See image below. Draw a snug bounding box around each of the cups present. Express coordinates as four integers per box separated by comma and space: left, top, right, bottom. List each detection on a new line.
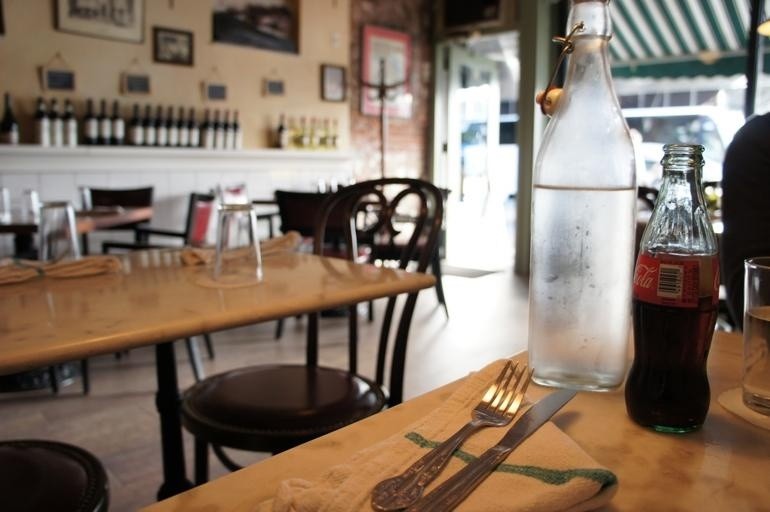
213, 204, 262, 288
37, 204, 80, 265
75, 185, 93, 213
1, 187, 40, 226
743, 257, 770, 418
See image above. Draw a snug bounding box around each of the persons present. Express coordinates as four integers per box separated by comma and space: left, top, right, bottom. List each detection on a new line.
719, 110, 770, 342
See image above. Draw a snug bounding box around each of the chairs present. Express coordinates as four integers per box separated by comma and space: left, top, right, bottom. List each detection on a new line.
174, 185, 446, 496
0, 436, 114, 512
3, 180, 446, 392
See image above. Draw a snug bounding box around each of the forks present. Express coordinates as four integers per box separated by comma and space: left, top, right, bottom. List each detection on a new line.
369, 355, 534, 512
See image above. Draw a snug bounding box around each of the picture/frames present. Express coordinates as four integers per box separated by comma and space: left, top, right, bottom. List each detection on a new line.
321, 64, 346, 101
201, 83, 227, 102
42, 66, 75, 92
123, 73, 152, 93
263, 79, 285, 95
58, 1, 145, 44
152, 26, 192, 65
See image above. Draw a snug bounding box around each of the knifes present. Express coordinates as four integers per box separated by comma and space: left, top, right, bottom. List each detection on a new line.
408, 388, 583, 512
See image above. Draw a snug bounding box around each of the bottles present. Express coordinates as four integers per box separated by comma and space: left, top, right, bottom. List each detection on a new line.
626, 145, 719, 439
1, 94, 341, 147
529, 0, 638, 396
